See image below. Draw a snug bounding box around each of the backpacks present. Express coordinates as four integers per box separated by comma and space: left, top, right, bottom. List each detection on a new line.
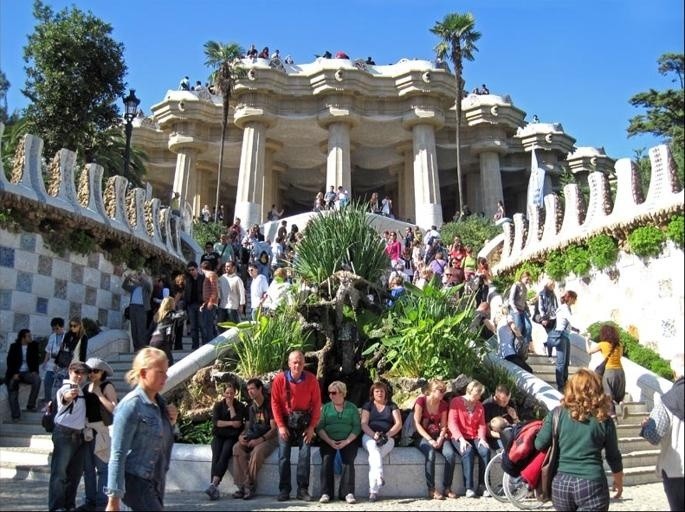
42, 383, 73, 432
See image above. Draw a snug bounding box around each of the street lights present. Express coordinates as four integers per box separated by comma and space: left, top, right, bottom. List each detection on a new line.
121, 88, 140, 182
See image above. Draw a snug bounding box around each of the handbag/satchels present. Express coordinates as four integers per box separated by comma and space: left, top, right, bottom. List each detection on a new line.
400, 411, 417, 447
547, 330, 561, 346
54, 349, 72, 368
533, 310, 546, 322
517, 342, 528, 360
541, 444, 557, 499
595, 358, 608, 376
100, 399, 120, 426
288, 411, 311, 428
216, 416, 243, 437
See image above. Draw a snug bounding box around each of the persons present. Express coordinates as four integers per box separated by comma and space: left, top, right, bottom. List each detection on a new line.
48, 346, 177, 512
122, 193, 313, 366
314, 50, 376, 66
452, 201, 506, 224
386, 224, 684, 512
532, 115, 539, 124
178, 76, 215, 93
314, 186, 412, 224
473, 83, 490, 94
246, 46, 294, 65
5, 317, 88, 422
204, 350, 402, 504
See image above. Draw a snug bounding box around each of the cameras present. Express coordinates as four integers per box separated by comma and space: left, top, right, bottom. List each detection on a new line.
377, 434, 387, 445
71, 384, 78, 389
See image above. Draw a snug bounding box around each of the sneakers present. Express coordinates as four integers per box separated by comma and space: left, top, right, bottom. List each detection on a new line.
319, 494, 329, 502
368, 494, 376, 502
466, 489, 475, 497
277, 491, 289, 501
232, 488, 253, 500
205, 485, 219, 500
444, 489, 456, 498
483, 490, 491, 496
429, 488, 442, 499
345, 493, 355, 504
297, 491, 311, 500
377, 478, 384, 488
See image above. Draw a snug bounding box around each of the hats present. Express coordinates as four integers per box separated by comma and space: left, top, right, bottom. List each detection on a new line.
85, 358, 113, 376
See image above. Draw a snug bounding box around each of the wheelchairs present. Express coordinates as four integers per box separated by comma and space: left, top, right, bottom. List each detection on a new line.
484, 421, 547, 510
